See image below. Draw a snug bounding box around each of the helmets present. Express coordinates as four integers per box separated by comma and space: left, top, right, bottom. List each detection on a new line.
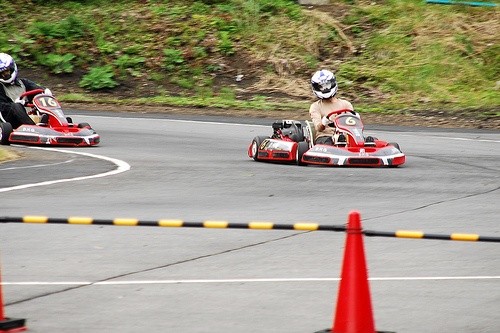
0, 51, 18, 84
312, 69, 339, 98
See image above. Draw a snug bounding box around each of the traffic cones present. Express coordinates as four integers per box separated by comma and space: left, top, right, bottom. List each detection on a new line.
311, 211, 396, 333
0, 257, 28, 333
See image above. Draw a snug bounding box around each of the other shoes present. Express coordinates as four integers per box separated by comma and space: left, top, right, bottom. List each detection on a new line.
366, 137, 374, 147
38, 113, 47, 127
66, 116, 72, 126
338, 135, 346, 147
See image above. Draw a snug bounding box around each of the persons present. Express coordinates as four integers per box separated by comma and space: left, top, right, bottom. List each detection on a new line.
0, 52, 73, 129
309, 69, 379, 146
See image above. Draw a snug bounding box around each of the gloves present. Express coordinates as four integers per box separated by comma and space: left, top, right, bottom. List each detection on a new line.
356, 112, 361, 118
321, 115, 329, 126
15, 97, 25, 105
42, 87, 52, 96
